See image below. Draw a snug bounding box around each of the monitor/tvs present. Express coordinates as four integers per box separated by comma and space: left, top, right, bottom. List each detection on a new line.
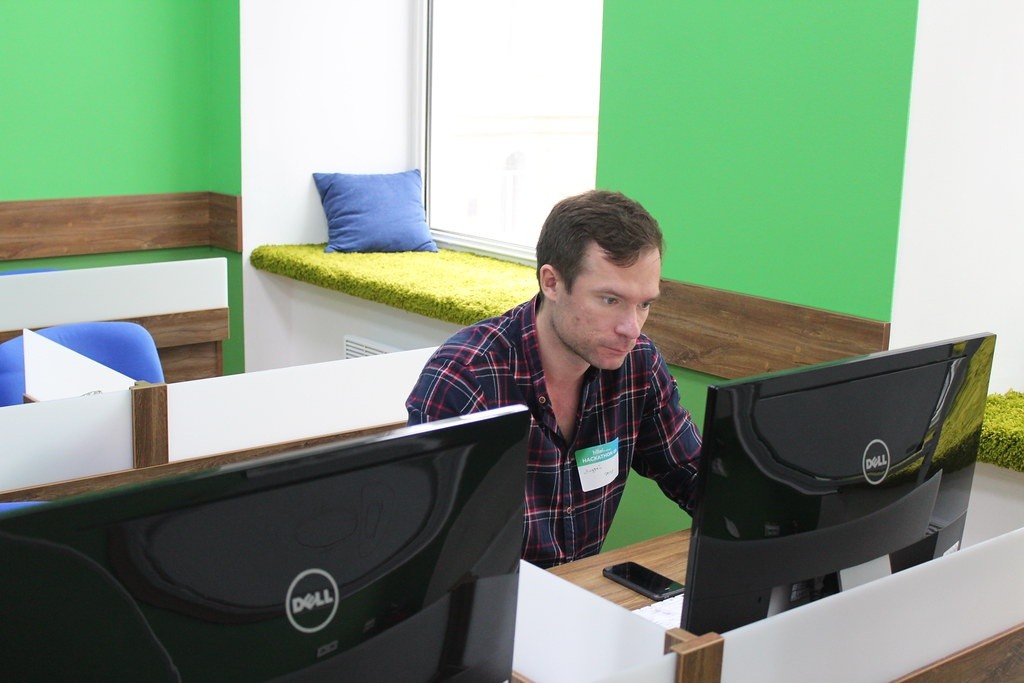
0, 403, 531, 683
678, 331, 997, 638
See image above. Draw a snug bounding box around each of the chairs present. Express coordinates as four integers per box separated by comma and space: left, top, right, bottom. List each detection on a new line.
1, 316, 169, 408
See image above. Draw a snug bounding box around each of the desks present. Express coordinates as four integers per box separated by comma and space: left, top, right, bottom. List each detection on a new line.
3, 335, 480, 509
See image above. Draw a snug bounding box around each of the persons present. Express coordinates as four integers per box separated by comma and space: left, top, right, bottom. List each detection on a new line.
400, 185, 706, 570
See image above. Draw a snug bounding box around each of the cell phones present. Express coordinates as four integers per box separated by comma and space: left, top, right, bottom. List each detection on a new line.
603, 561, 685, 602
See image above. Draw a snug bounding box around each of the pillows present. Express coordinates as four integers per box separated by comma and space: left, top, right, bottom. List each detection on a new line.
311, 166, 440, 258
